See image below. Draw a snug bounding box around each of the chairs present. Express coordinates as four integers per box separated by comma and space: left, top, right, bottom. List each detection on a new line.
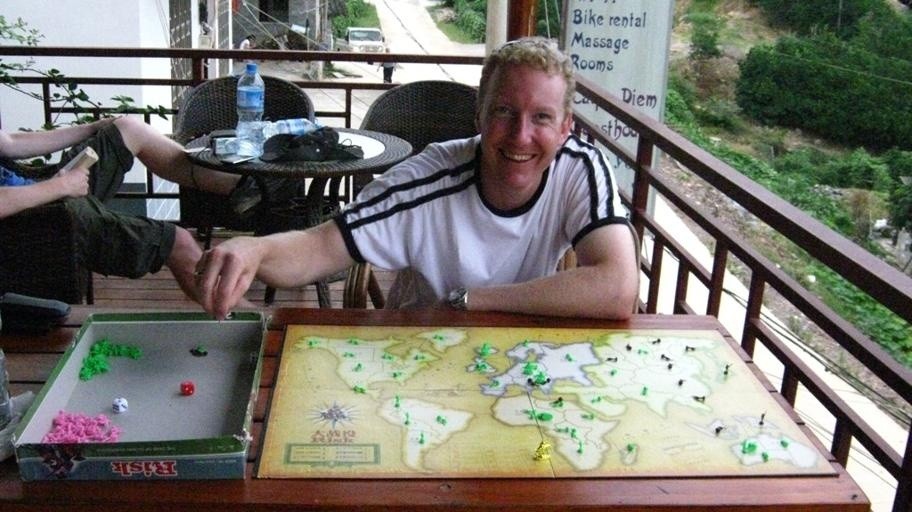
174, 75, 314, 248
351, 79, 477, 202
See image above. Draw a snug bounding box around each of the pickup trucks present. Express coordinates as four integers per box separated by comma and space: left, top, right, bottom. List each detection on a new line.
335, 27, 385, 53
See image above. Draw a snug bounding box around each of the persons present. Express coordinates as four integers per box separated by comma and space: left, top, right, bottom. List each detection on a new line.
1, 111, 260, 310
1, 312, 35, 462
187, 37, 647, 323
375, 47, 396, 82
239, 34, 256, 62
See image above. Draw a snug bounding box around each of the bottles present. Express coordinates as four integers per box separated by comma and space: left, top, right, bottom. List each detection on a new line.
235, 63, 265, 157
262, 118, 323, 144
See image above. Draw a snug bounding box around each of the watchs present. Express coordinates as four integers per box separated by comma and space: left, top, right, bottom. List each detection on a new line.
448, 287, 468, 310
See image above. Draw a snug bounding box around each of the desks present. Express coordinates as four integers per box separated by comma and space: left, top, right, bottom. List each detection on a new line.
182, 126, 413, 308
1, 305, 869, 509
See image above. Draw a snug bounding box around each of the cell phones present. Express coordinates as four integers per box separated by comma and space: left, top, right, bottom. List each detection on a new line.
220, 155, 255, 164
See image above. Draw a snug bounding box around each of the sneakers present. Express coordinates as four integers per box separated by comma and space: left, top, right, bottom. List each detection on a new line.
228, 175, 288, 217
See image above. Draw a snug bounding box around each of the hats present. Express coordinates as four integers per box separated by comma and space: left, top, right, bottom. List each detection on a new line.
258, 127, 365, 162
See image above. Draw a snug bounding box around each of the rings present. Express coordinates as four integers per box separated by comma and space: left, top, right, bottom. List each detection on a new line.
193, 268, 205, 279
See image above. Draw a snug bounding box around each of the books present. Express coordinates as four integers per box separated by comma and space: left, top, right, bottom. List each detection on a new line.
51, 146, 98, 179
